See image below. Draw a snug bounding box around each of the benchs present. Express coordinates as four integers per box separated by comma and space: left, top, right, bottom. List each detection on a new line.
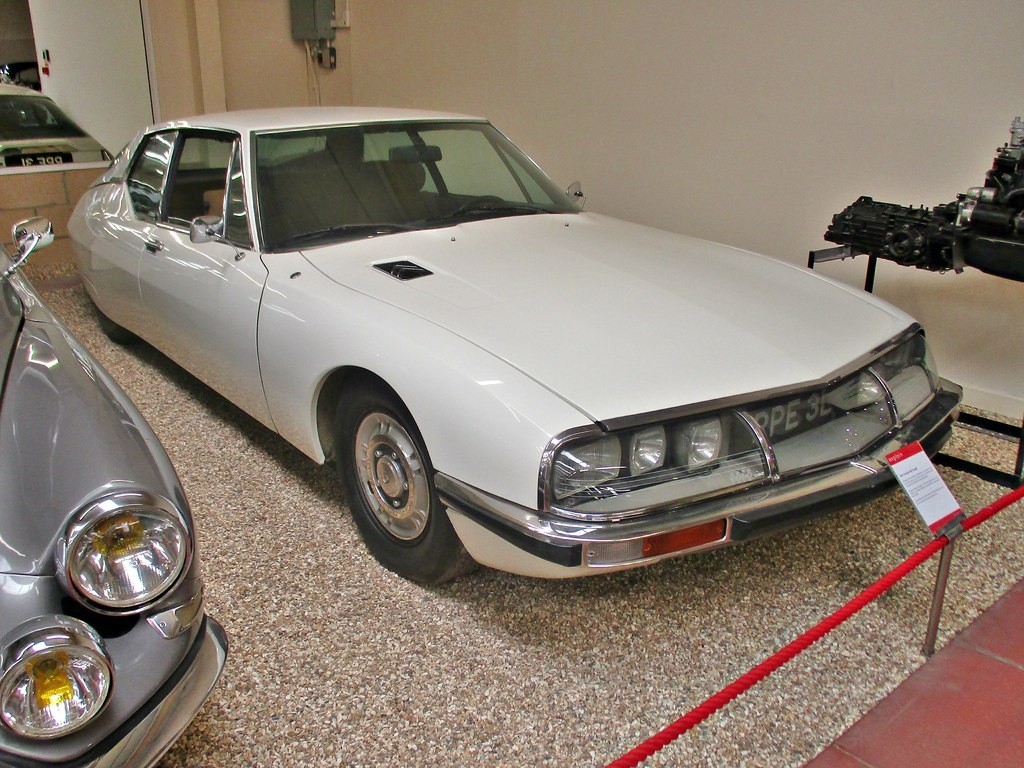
204, 159, 433, 246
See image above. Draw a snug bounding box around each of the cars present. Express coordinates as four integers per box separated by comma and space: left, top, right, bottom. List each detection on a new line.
67, 107, 965, 587
0, 83, 115, 167
0, 216, 228, 768
